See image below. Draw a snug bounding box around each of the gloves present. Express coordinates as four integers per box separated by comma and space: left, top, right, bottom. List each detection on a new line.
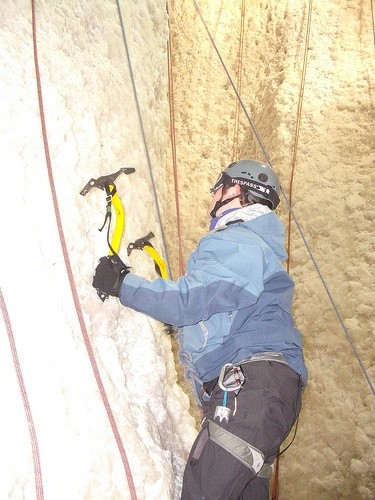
93, 256, 122, 297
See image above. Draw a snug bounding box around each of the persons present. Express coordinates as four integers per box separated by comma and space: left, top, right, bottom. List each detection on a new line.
92, 159, 308, 500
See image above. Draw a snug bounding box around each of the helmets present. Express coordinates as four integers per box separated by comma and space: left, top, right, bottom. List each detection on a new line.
211, 160, 280, 207
